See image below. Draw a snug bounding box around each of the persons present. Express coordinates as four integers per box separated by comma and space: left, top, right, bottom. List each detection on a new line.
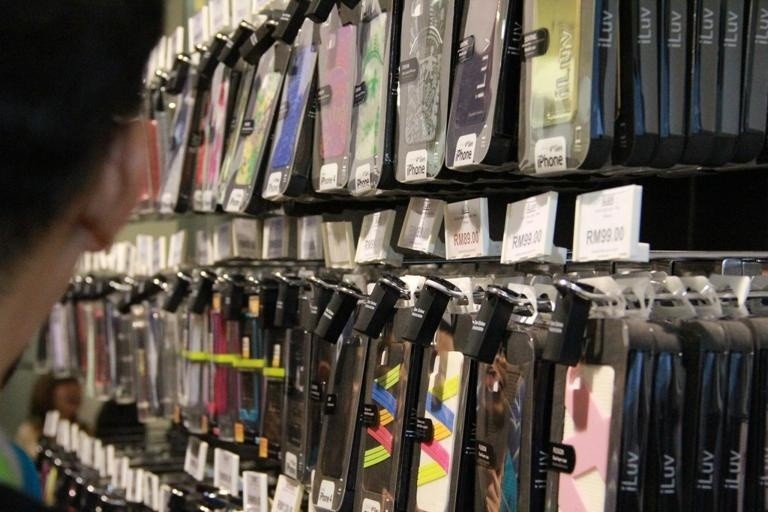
0, 0, 163, 511
